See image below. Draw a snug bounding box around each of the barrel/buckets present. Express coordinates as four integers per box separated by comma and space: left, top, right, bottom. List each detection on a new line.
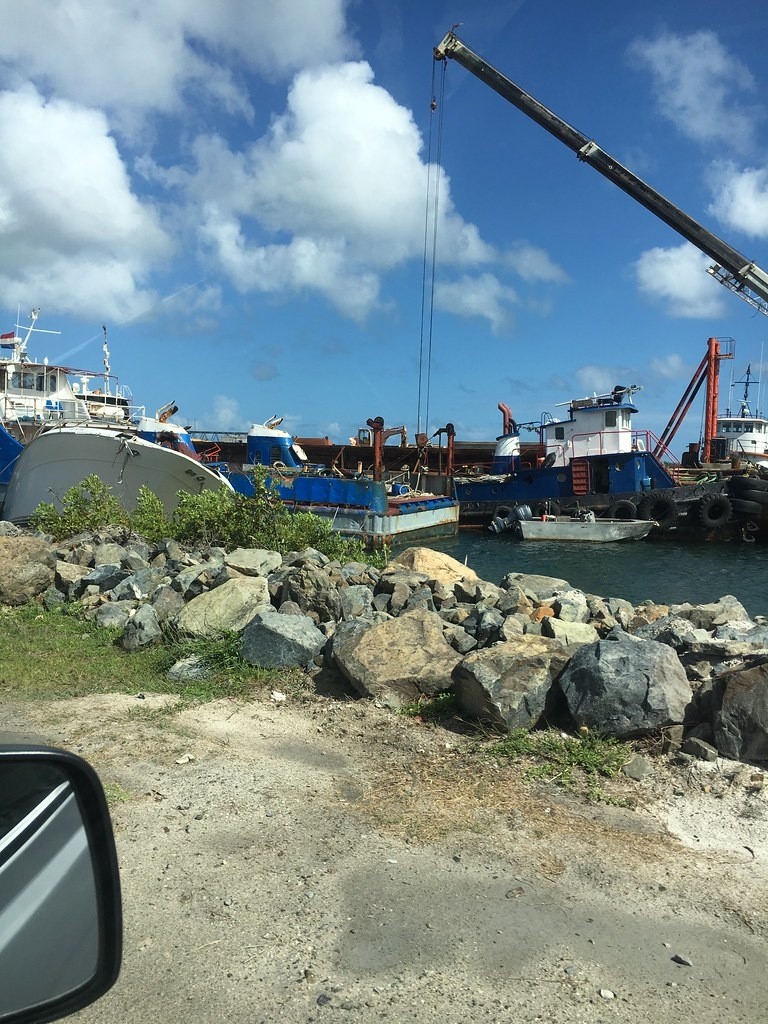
401, 483, 409, 494
732, 458, 740, 469
391, 484, 401, 496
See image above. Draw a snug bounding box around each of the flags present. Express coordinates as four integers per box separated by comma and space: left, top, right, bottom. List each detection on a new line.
0, 332, 15, 349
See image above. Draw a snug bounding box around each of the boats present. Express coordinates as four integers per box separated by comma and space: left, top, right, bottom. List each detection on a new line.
214, 415, 461, 551
700, 360, 768, 475
488, 505, 656, 544
452, 382, 732, 533
0, 418, 238, 527
0, 300, 221, 511
62, 322, 548, 471
